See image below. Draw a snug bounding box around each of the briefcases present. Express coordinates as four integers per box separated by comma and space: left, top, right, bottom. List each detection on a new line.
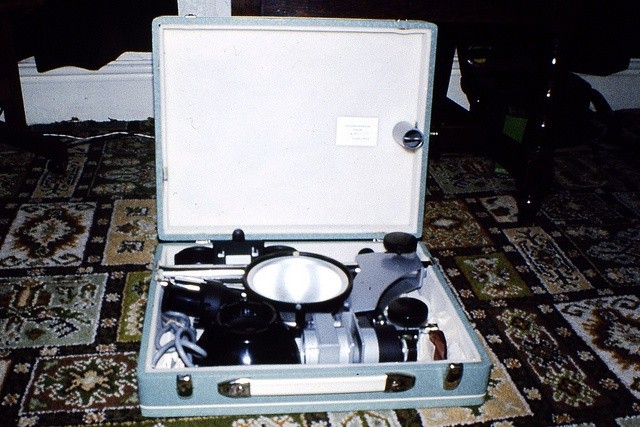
138, 14, 492, 418
